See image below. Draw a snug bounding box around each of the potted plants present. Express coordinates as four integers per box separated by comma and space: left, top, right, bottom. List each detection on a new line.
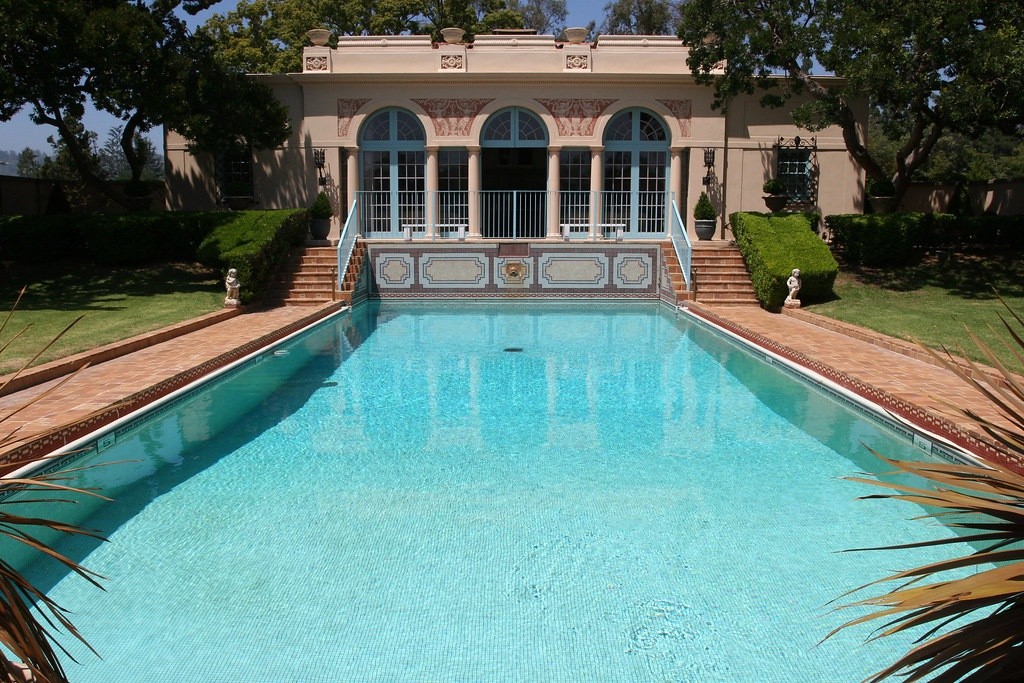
307, 190, 334, 240
762, 179, 789, 213
694, 191, 717, 241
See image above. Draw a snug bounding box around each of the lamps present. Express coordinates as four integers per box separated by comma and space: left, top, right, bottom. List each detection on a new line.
702, 148, 714, 185
616, 230, 623, 240
314, 148, 326, 185
458, 226, 465, 240
404, 227, 413, 241
562, 224, 570, 242
